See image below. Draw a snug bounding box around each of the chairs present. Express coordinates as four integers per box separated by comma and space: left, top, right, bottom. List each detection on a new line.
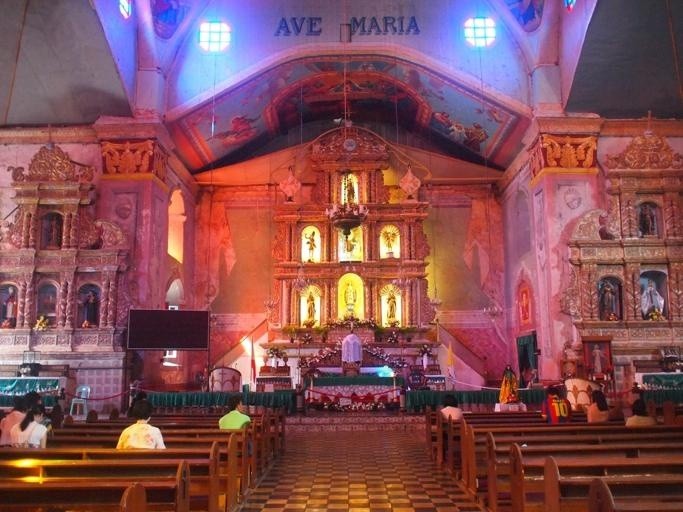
70, 385, 91, 414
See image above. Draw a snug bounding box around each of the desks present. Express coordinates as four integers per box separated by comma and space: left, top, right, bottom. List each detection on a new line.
402, 387, 564, 412
300, 375, 404, 411
144, 390, 298, 416
0, 376, 67, 410
640, 373, 683, 402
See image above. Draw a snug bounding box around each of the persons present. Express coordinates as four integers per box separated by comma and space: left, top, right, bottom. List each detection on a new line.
542, 385, 656, 427
114, 402, 160, 452
218, 396, 253, 457
0, 391, 55, 449
439, 396, 463, 421
126, 392, 147, 416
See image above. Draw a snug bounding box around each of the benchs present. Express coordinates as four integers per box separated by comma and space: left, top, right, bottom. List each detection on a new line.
417, 402, 683, 512
0, 405, 285, 510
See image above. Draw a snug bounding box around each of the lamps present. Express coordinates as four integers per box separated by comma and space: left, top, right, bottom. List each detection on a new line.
389, 1, 411, 294
259, 1, 288, 315
462, 2, 505, 324
327, 1, 370, 239
425, 2, 445, 309
289, 1, 314, 300
